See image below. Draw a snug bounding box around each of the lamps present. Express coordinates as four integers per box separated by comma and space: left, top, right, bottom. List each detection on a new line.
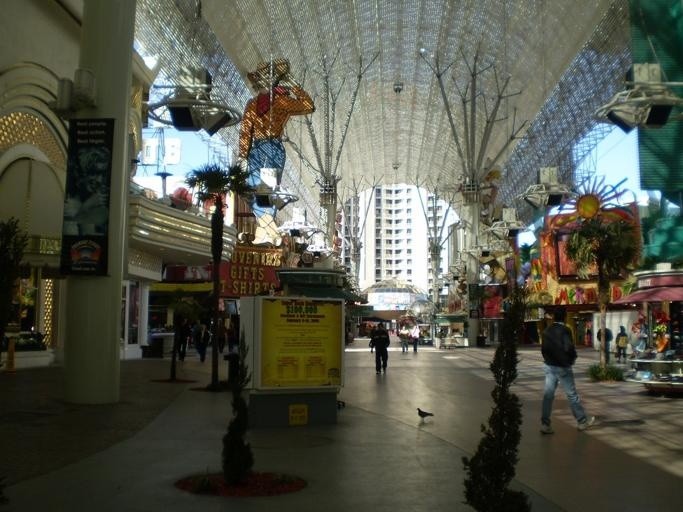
145, 66, 242, 136
245, 180, 334, 260
448, 165, 579, 283
47, 65, 99, 115
594, 61, 683, 134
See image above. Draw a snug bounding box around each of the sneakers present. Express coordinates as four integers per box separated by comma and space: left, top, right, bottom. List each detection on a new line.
539, 423, 553, 434
577, 416, 595, 431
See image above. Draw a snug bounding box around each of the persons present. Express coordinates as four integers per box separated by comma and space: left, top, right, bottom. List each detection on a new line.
597, 327, 613, 363
177, 318, 236, 365
370, 325, 377, 353
369, 322, 390, 375
399, 325, 409, 353
238, 56, 316, 247
631, 313, 683, 359
408, 325, 420, 352
615, 325, 630, 364
62, 142, 111, 236
540, 309, 597, 435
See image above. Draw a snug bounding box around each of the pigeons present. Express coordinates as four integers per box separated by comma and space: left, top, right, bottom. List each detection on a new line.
416, 408, 434, 421
